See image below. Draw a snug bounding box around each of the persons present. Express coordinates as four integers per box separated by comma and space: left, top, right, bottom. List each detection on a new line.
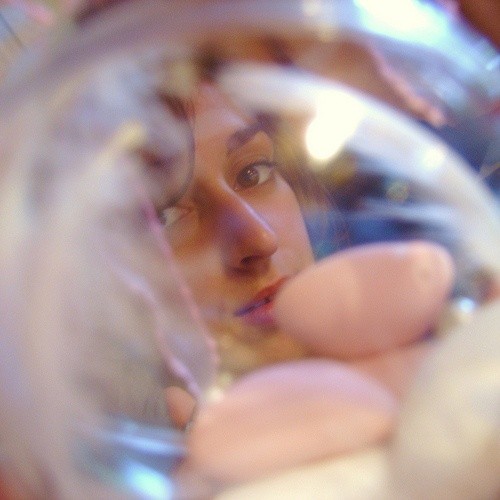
33, 51, 500, 500
